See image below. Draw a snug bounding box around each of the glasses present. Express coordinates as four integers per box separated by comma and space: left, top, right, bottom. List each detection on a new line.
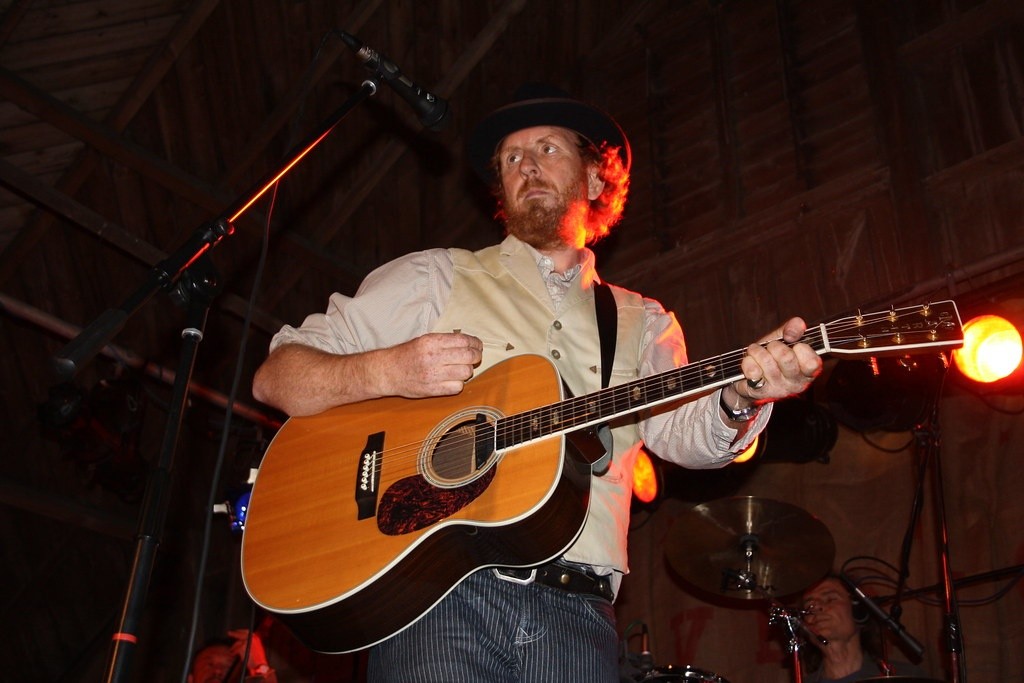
800, 592, 860, 610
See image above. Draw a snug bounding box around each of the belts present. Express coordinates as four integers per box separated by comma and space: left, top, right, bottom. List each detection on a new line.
492, 557, 614, 603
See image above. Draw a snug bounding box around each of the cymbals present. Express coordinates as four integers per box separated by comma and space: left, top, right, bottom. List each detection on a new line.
659, 497, 837, 601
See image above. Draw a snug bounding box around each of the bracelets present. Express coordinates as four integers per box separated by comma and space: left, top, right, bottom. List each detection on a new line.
249, 664, 271, 676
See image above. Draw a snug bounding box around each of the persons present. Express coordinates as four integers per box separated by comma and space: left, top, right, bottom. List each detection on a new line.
799, 568, 931, 683
187, 627, 278, 683
252, 85, 825, 683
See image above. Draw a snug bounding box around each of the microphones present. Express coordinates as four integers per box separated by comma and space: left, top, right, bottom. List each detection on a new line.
639, 624, 654, 672
335, 30, 452, 129
842, 574, 925, 657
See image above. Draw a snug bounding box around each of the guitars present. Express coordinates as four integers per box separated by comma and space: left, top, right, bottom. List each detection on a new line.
238, 301, 968, 659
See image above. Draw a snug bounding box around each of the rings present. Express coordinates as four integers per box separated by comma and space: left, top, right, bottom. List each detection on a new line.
747, 378, 766, 389
806, 376, 816, 381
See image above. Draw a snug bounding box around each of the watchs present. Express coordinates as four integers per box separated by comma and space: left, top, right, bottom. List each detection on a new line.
720, 396, 757, 422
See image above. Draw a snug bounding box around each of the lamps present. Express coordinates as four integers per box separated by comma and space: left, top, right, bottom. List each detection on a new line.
632, 314, 1024, 506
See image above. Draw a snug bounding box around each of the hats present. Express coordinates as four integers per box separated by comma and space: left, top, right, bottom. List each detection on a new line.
465, 85, 631, 226
